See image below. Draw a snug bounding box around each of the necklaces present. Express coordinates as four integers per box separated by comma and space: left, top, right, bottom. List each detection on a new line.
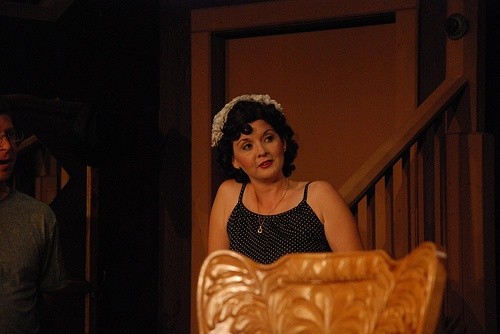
257, 178, 289, 233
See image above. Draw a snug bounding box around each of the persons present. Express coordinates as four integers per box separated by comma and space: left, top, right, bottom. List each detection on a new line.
0, 99, 65, 334
208, 94, 365, 264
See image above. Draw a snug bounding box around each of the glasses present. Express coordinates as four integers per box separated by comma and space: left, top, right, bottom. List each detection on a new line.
1, 129, 24, 143
